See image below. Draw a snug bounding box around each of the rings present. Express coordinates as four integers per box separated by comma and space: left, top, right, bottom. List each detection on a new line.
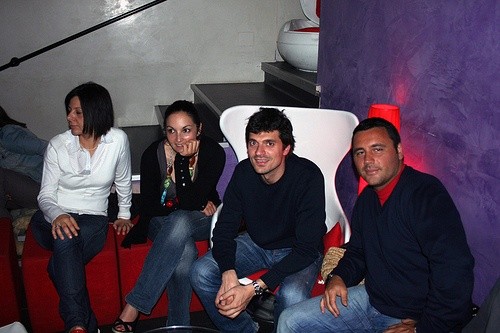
55, 225, 60, 228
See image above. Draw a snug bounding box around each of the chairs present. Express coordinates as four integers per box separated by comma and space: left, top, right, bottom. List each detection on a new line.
209, 106, 361, 298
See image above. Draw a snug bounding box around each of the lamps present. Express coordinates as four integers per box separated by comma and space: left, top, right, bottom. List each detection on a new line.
369, 104, 401, 134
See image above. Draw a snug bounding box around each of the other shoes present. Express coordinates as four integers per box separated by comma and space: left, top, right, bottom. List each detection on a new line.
70, 327, 85, 333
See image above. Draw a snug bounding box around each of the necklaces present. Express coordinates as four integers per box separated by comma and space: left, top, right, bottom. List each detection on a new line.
161, 149, 196, 209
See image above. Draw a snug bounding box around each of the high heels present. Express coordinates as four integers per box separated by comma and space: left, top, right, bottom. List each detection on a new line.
112, 311, 140, 333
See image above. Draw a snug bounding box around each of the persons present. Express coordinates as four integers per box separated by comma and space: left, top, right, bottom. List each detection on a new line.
30, 82, 134, 333
112, 100, 226, 333
0, 106, 48, 208
189, 107, 326, 333
276, 117, 475, 333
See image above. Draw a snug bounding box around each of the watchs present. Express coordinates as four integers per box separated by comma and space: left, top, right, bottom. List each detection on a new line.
252, 280, 263, 296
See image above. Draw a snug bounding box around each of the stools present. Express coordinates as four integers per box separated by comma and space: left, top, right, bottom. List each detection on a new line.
0, 214, 210, 333
